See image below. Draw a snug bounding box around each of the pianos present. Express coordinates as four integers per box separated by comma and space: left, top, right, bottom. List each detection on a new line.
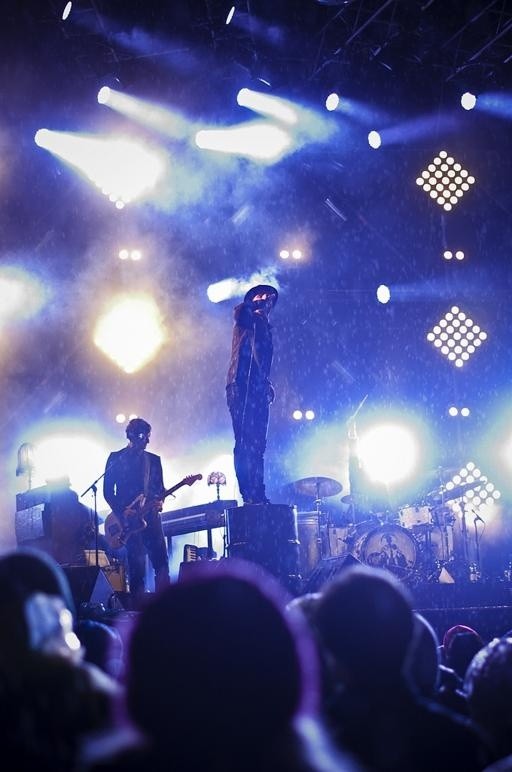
184, 544, 217, 562
160, 500, 238, 536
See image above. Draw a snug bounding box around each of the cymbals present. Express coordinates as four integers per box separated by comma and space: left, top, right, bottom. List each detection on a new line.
293, 477, 343, 497
437, 481, 486, 505
341, 494, 360, 505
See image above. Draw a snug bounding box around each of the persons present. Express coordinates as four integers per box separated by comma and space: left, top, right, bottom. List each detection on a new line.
344, 415, 393, 524
0, 547, 342, 772
224, 285, 278, 506
101, 418, 170, 593
316, 565, 510, 771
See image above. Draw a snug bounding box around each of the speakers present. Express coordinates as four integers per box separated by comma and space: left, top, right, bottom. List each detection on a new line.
14, 484, 87, 565
299, 554, 364, 594
62, 565, 126, 608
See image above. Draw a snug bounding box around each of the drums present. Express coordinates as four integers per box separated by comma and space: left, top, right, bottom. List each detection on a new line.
352, 522, 420, 582
397, 503, 435, 534
327, 523, 354, 563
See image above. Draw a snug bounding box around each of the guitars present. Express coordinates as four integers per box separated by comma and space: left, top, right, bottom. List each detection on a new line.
104, 474, 202, 549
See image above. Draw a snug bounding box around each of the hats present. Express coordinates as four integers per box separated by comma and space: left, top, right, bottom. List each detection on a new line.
243, 283, 280, 307
241, 494, 273, 507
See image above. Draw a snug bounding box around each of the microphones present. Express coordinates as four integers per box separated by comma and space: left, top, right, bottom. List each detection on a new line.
471, 510, 485, 524
266, 293, 276, 301
